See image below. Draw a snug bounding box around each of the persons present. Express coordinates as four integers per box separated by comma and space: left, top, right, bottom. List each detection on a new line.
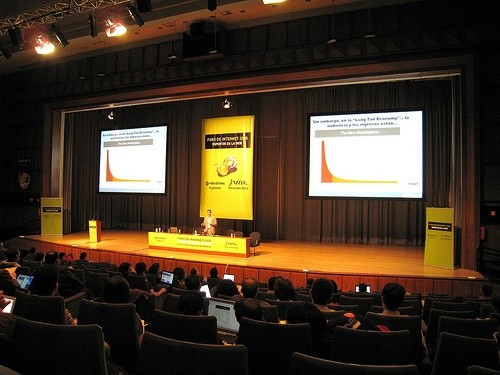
200, 210, 217, 234
0, 240, 500, 361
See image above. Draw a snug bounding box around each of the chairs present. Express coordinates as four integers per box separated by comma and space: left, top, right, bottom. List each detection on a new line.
0, 260, 500, 375
195, 227, 261, 258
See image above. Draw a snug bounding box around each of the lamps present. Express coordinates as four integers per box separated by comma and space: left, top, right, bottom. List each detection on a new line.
89, 10, 97, 38
0, 38, 12, 58
108, 110, 116, 119
48, 22, 69, 48
8, 24, 27, 49
222, 97, 232, 109
34, 30, 53, 55
105, 0, 152, 38
208, 0, 218, 11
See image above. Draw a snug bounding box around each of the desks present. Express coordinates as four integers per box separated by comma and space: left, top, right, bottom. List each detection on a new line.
148, 232, 250, 257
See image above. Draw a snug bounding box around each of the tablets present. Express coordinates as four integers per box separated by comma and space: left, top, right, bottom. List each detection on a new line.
2, 295, 16, 315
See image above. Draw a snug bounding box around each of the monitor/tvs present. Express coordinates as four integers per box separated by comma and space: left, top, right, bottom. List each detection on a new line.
208, 297, 240, 333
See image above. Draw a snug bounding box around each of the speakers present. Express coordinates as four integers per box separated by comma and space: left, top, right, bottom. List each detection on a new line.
190, 20, 211, 55
0, 28, 23, 55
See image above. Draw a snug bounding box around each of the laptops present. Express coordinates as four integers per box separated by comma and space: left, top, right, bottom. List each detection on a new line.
16, 274, 34, 290
355, 285, 371, 293
224, 273, 235, 282
157, 270, 175, 287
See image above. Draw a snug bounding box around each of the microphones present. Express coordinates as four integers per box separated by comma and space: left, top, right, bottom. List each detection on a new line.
92, 214, 96, 221
